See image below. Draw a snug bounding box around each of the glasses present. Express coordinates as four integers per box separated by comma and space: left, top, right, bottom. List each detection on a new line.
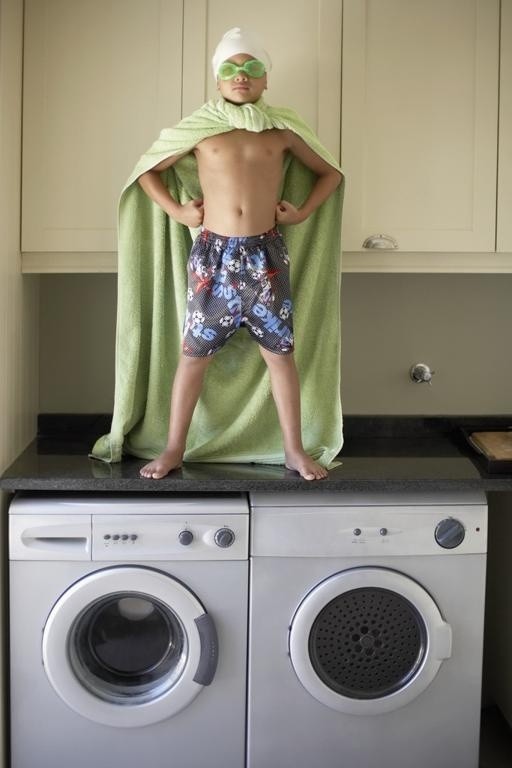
218, 59, 265, 80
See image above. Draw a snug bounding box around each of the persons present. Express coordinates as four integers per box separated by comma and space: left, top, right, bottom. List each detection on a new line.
136, 25, 345, 482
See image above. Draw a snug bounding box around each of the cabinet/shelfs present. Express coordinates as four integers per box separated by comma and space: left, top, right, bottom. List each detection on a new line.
20, 1, 340, 275
344, 1, 512, 276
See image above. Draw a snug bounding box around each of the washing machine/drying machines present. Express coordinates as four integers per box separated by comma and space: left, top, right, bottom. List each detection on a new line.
8, 490, 249, 768
247, 493, 488, 768
